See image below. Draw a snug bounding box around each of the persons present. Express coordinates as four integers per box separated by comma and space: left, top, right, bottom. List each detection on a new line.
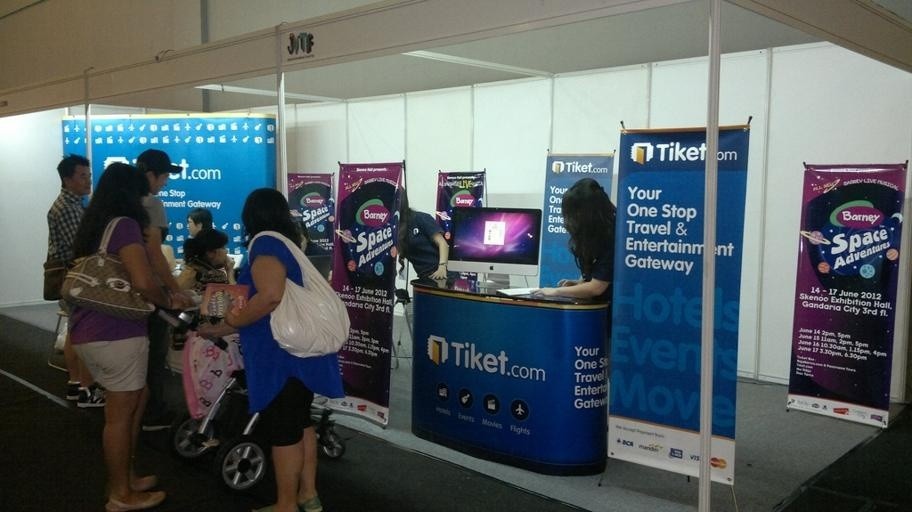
530, 178, 616, 298
298, 222, 332, 278
46, 154, 93, 302
160, 205, 237, 290
135, 149, 183, 430
398, 187, 450, 281
73, 161, 189, 510
200, 189, 345, 511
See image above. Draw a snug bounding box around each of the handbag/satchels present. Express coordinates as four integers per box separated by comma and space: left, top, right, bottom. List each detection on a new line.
44, 261, 65, 300
61, 254, 155, 320
182, 331, 243, 420
270, 278, 350, 358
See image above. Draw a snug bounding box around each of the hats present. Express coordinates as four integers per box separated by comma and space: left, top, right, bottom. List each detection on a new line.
137, 150, 182, 173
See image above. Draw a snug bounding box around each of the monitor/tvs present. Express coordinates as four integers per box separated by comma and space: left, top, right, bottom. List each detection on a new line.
444, 206, 541, 288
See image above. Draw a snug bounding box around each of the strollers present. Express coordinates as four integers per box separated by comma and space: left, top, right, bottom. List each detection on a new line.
154, 298, 348, 492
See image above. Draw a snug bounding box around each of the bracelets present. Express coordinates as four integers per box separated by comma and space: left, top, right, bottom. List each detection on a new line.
437, 260, 446, 267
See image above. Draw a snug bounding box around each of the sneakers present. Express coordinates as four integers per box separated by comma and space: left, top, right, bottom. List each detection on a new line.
106, 476, 165, 512
67, 380, 108, 408
